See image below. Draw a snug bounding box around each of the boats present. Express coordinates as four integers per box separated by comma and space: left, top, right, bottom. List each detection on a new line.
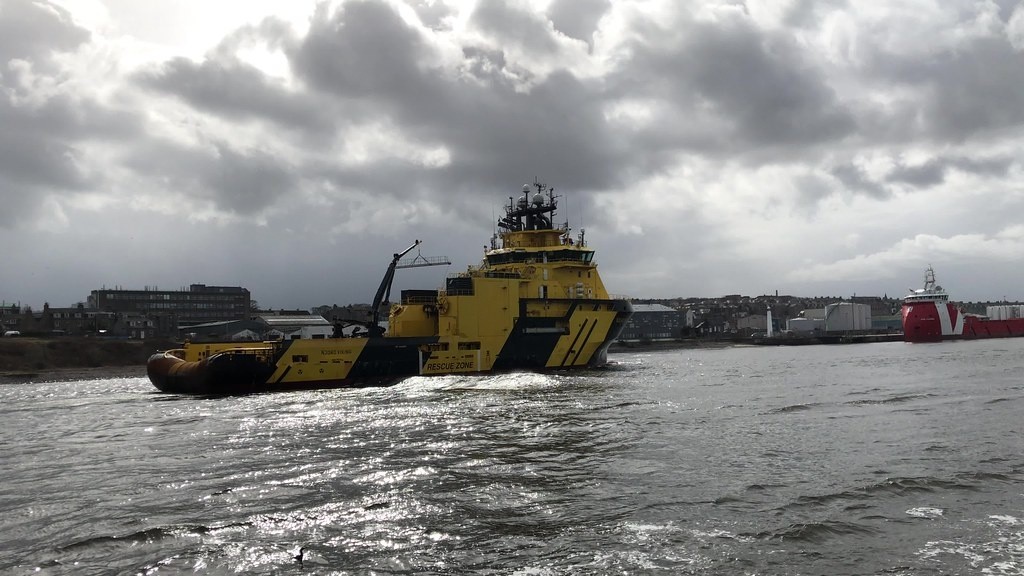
148, 178, 640, 397
901, 267, 1024, 344
